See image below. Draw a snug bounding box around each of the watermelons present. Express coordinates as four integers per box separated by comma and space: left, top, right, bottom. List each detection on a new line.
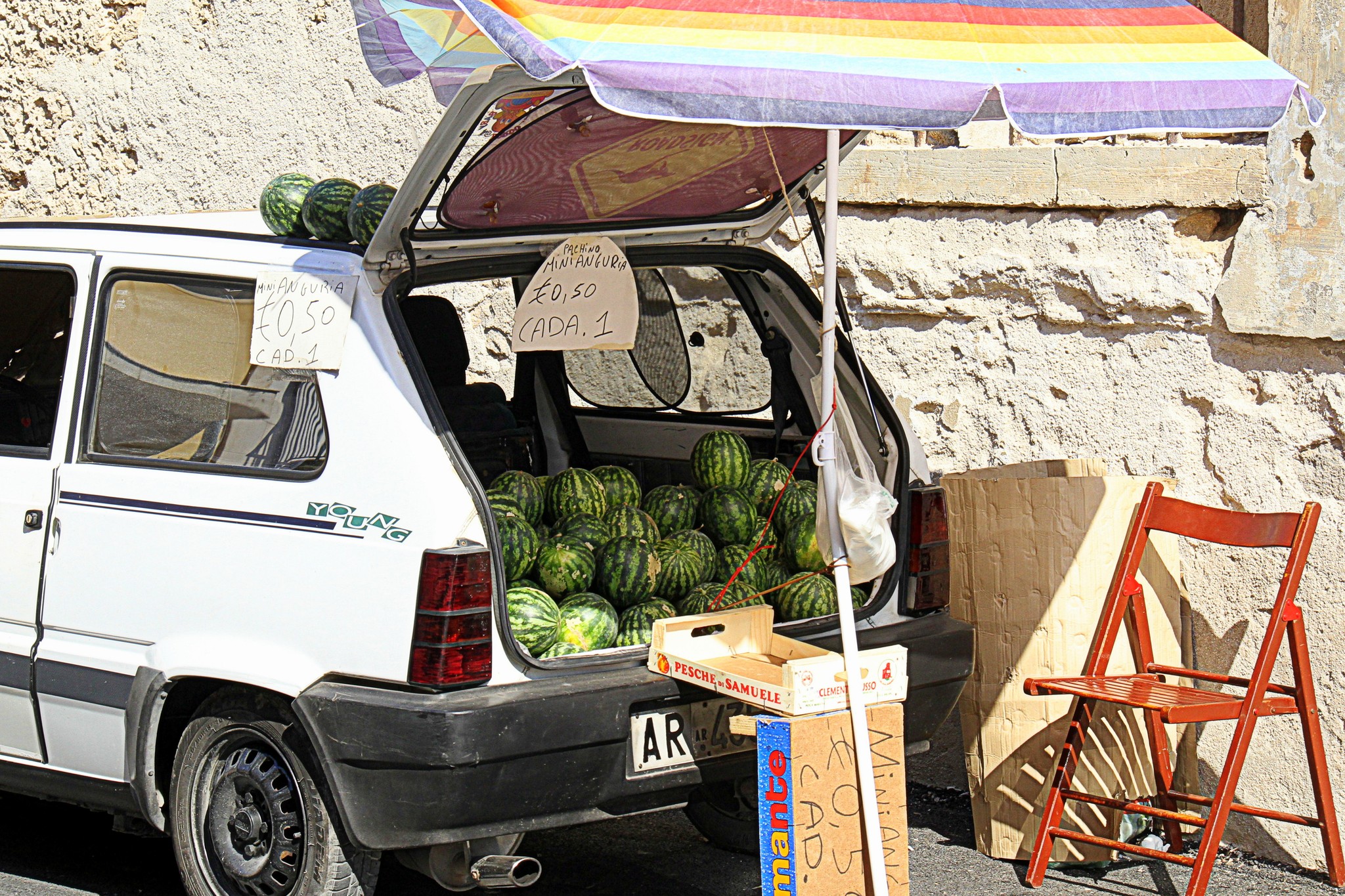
347, 184, 398, 248
301, 178, 364, 243
484, 427, 869, 658
259, 172, 319, 239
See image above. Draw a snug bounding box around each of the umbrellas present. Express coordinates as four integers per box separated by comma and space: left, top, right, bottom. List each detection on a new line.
351, 0, 1327, 896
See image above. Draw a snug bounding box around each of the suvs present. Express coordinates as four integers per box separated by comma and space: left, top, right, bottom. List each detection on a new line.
0, 58, 980, 895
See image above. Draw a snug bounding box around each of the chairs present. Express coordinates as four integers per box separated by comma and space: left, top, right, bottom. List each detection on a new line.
398, 295, 533, 489
1023, 482, 1345, 896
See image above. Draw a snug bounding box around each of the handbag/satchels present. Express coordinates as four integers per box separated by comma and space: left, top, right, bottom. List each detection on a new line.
810, 351, 898, 586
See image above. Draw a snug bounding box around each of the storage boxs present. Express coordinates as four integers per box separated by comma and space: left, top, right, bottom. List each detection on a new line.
726, 703, 909, 896
649, 603, 908, 717
